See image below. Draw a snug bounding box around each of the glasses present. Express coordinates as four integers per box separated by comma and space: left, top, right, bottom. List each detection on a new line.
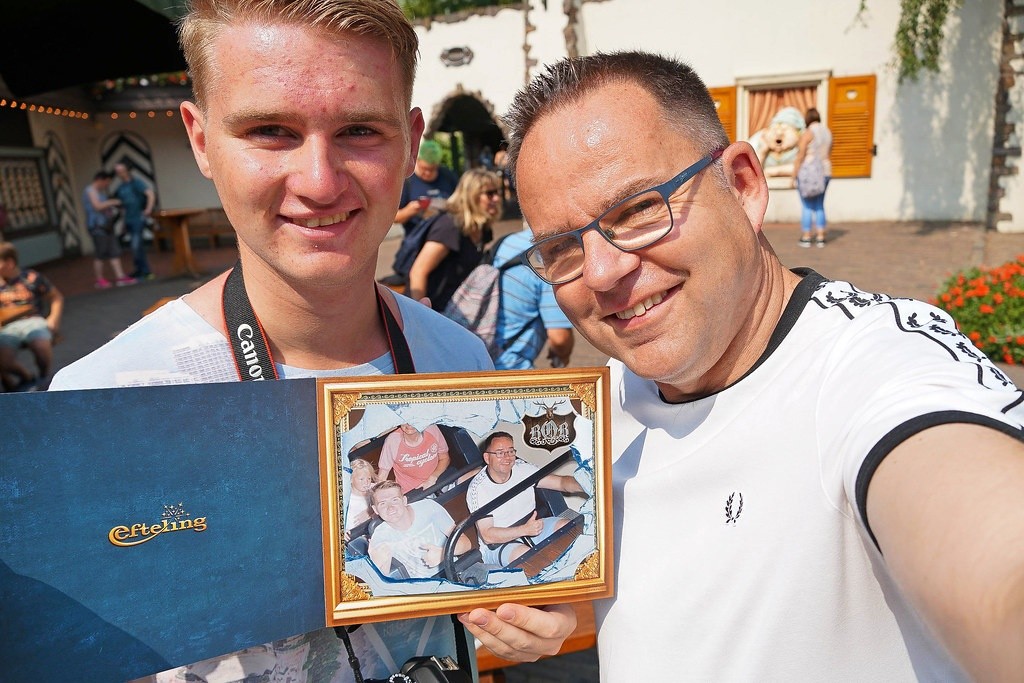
479, 189, 497, 199
521, 150, 728, 286
483, 449, 517, 458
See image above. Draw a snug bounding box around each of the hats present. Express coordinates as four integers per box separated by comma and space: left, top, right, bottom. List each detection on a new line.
420, 140, 444, 166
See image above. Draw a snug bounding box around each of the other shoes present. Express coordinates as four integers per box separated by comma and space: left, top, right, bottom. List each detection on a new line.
95, 279, 112, 289
129, 272, 156, 280
114, 278, 138, 288
816, 237, 825, 248
798, 237, 812, 248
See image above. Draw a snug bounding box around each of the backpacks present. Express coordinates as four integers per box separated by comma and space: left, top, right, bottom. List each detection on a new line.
439, 232, 542, 366
390, 211, 468, 280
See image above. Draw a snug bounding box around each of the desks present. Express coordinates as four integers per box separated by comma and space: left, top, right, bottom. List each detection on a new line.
160, 208, 207, 283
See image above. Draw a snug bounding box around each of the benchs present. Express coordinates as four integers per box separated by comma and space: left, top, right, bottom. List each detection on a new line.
154, 205, 231, 255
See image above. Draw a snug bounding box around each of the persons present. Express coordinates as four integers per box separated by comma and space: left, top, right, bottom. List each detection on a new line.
378, 422, 459, 499
0, 242, 66, 393
790, 107, 833, 248
465, 431, 593, 567
479, 225, 575, 372
393, 141, 460, 238
343, 459, 407, 543
401, 166, 504, 313
42, 0, 579, 683
83, 169, 137, 290
367, 480, 471, 580
109, 161, 159, 281
499, 47, 1024, 683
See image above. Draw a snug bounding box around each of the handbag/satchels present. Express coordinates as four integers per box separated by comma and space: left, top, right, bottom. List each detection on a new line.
798, 158, 826, 199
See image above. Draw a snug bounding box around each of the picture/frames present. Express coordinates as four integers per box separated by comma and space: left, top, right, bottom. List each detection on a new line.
315, 365, 614, 628
